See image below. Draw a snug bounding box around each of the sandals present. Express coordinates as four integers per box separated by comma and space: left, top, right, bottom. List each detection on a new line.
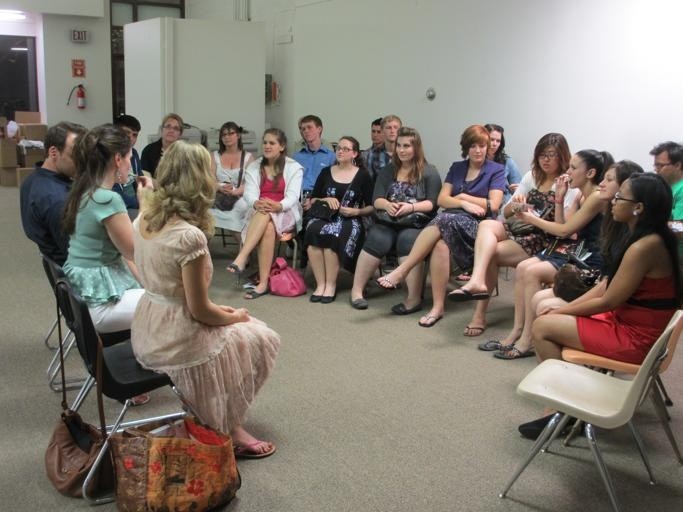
244, 288, 268, 299
227, 259, 250, 273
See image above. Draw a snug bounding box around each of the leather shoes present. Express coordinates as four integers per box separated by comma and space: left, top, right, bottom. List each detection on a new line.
350, 295, 367, 308
519, 412, 565, 437
321, 296, 335, 302
311, 295, 321, 301
392, 303, 422, 314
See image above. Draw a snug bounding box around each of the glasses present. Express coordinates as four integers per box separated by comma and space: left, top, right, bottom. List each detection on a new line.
654, 162, 673, 167
335, 146, 354, 151
221, 131, 237, 136
614, 191, 639, 203
162, 124, 182, 132
539, 151, 560, 158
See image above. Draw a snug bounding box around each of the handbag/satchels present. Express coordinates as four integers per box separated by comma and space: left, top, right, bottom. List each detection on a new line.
308, 200, 338, 220
441, 199, 493, 219
378, 205, 431, 227
213, 149, 246, 211
110, 416, 241, 512
551, 263, 601, 301
268, 256, 306, 296
45, 336, 115, 499
505, 204, 540, 232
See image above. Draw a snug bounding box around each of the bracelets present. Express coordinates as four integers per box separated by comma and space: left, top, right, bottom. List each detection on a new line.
412, 203, 416, 213
279, 202, 284, 213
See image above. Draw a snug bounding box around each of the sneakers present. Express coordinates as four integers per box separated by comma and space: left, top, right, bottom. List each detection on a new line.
302, 265, 314, 281
285, 245, 297, 258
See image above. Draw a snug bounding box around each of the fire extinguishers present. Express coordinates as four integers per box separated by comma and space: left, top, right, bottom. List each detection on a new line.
67, 84, 88, 109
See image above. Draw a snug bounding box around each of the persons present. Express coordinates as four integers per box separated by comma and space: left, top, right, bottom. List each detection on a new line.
359, 117, 384, 155
530, 160, 644, 318
19, 121, 89, 266
363, 115, 402, 180
226, 128, 304, 300
62, 124, 155, 336
374, 124, 506, 327
350, 127, 442, 316
130, 140, 279, 459
484, 123, 522, 206
517, 170, 683, 440
112, 114, 142, 209
285, 114, 336, 269
478, 149, 615, 360
303, 136, 374, 304
448, 132, 582, 337
649, 141, 683, 223
141, 112, 185, 178
209, 121, 258, 269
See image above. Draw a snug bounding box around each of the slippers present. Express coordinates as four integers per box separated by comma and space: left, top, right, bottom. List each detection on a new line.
232, 436, 276, 457
129, 392, 151, 404
457, 271, 470, 279
418, 314, 442, 327
495, 347, 536, 360
464, 325, 484, 335
377, 274, 402, 291
447, 287, 489, 301
479, 339, 511, 351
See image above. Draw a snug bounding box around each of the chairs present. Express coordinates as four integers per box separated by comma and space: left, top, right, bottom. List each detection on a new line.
498, 310, 683, 512
541, 316, 683, 465
48, 279, 141, 436
598, 367, 673, 421
55, 281, 187, 506
42, 256, 88, 385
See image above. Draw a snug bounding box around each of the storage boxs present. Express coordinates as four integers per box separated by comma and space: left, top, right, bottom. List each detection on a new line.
16, 168, 36, 188
0, 165, 20, 186
18, 145, 45, 167
15, 112, 48, 141
0, 139, 18, 168
0, 117, 8, 137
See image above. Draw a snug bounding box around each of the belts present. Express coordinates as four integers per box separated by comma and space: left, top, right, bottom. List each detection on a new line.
303, 189, 312, 194
